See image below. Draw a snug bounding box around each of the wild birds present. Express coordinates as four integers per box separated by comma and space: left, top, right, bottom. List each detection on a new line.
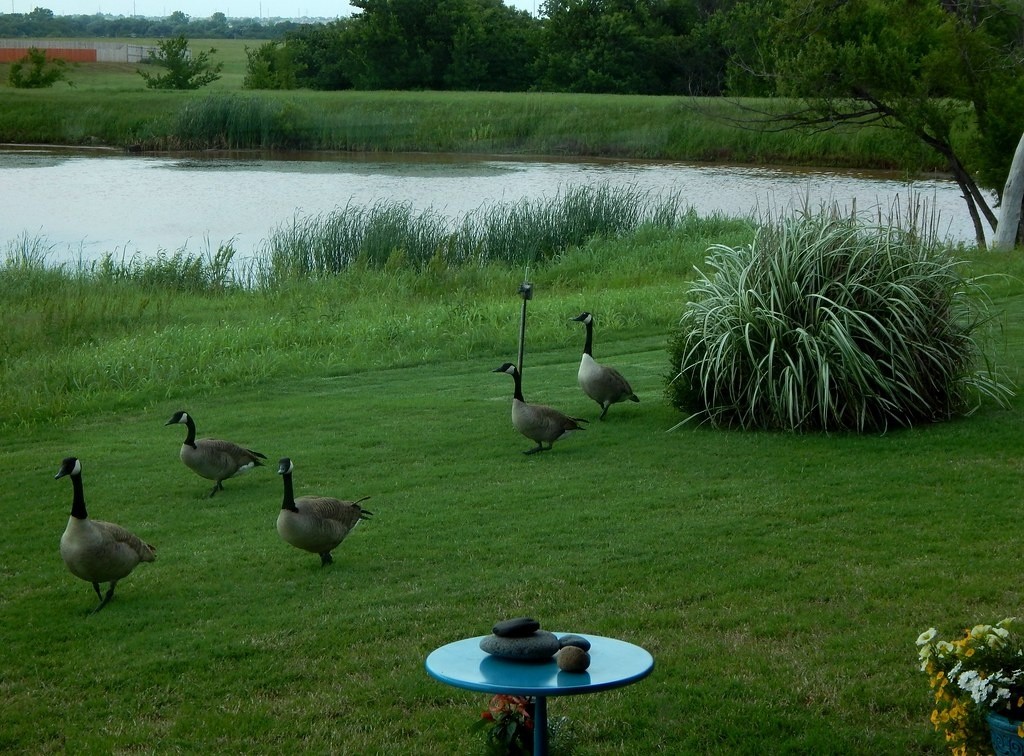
492, 362, 590, 457
55, 457, 157, 611
165, 410, 268, 497
276, 457, 374, 566
570, 310, 640, 421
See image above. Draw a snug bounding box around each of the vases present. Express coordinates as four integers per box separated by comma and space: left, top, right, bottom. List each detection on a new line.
987, 709, 1024, 756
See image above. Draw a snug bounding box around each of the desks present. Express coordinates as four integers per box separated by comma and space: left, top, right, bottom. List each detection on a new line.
424, 631, 655, 756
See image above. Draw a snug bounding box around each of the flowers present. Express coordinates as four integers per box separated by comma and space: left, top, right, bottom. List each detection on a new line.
914, 616, 1024, 756
